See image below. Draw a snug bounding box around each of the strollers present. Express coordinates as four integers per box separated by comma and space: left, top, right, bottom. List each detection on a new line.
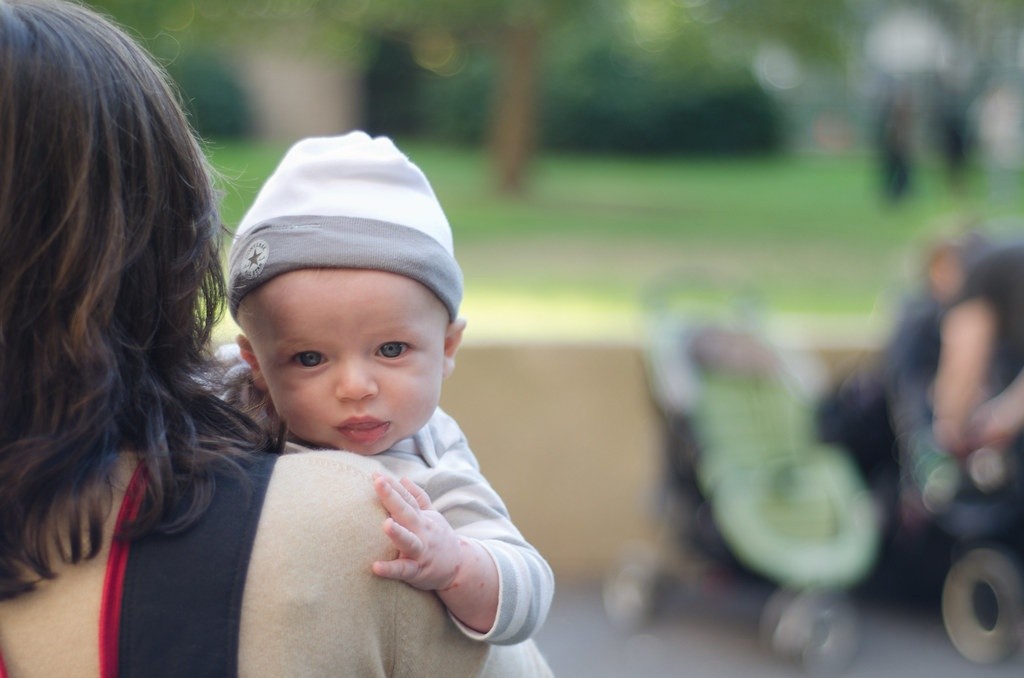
613, 305, 910, 675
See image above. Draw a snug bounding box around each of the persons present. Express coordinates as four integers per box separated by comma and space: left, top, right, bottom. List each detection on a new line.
0, 2, 557, 678
201, 130, 557, 648
703, 232, 1023, 634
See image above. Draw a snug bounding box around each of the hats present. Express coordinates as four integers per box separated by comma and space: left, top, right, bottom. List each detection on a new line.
228, 130, 464, 323
